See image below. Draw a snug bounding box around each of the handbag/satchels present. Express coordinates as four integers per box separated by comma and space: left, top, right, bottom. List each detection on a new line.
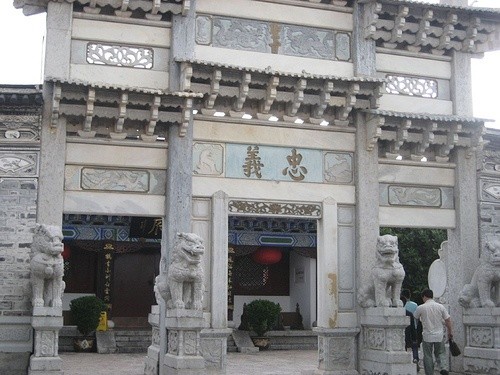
448, 338, 461, 357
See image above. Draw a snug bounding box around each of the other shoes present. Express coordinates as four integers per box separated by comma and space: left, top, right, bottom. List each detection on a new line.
413, 359, 420, 372
441, 370, 448, 375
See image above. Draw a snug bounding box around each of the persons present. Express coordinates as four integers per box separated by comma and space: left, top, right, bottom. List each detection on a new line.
413, 289, 453, 375
402, 289, 419, 329
399, 296, 421, 371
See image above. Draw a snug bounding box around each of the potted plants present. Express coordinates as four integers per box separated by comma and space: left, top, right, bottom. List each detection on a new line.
241, 299, 281, 350
67, 296, 109, 352
